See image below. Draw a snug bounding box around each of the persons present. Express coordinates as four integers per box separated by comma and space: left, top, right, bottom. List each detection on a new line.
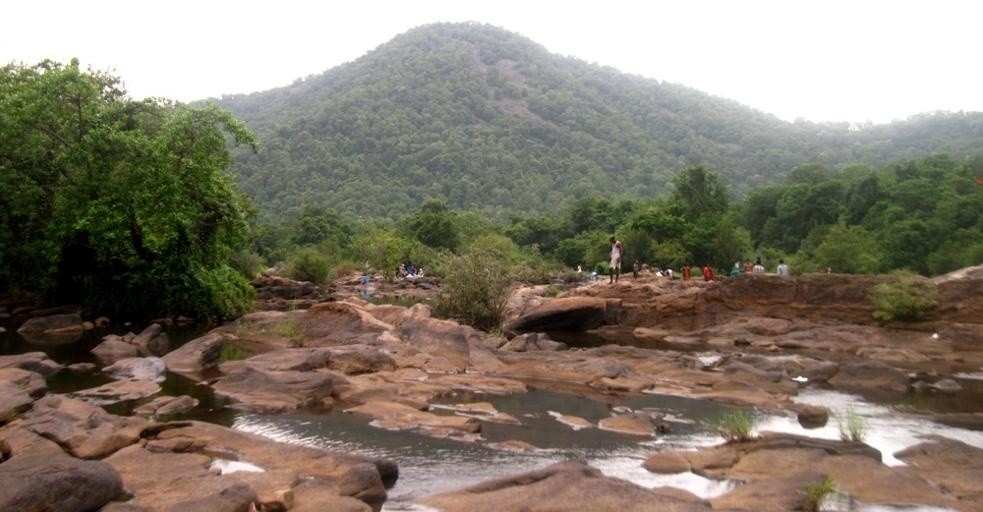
575, 263, 582, 273
396, 261, 425, 279
632, 256, 792, 280
606, 234, 623, 286
